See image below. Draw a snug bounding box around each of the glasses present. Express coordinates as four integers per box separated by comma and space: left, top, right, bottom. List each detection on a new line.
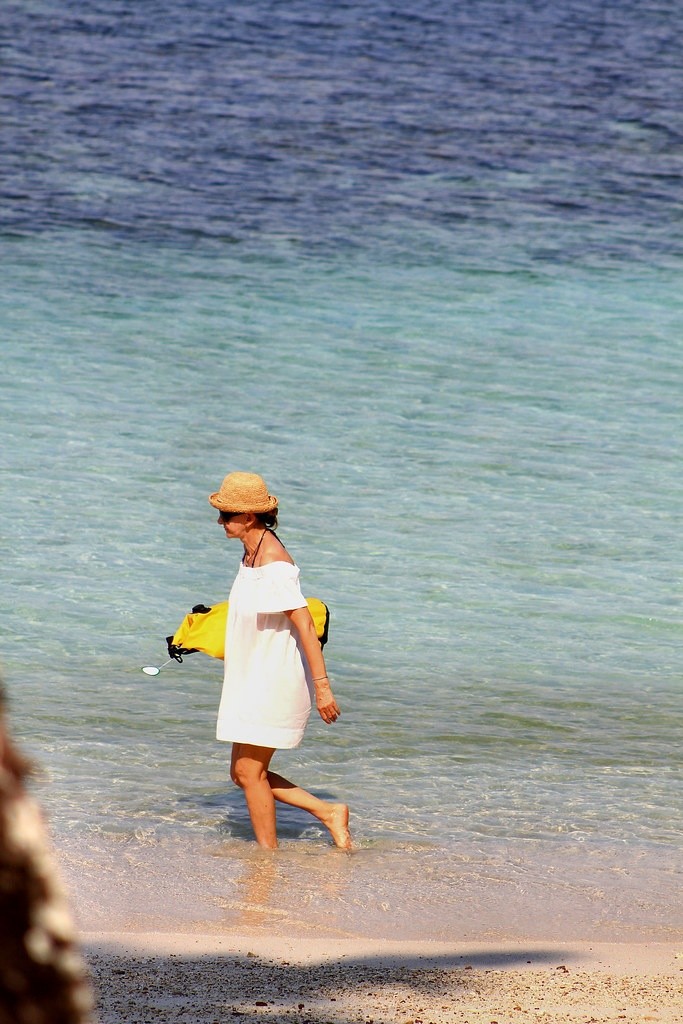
219, 511, 246, 523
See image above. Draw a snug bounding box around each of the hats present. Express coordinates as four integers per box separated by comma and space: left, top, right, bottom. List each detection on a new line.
209, 472, 279, 514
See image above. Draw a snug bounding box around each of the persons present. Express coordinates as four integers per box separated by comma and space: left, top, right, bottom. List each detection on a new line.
209, 472, 353, 849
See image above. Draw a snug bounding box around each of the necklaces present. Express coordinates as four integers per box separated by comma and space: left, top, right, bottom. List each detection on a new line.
245, 548, 256, 562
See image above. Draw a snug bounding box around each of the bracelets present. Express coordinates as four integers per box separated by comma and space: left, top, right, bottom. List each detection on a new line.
312, 676, 328, 681
